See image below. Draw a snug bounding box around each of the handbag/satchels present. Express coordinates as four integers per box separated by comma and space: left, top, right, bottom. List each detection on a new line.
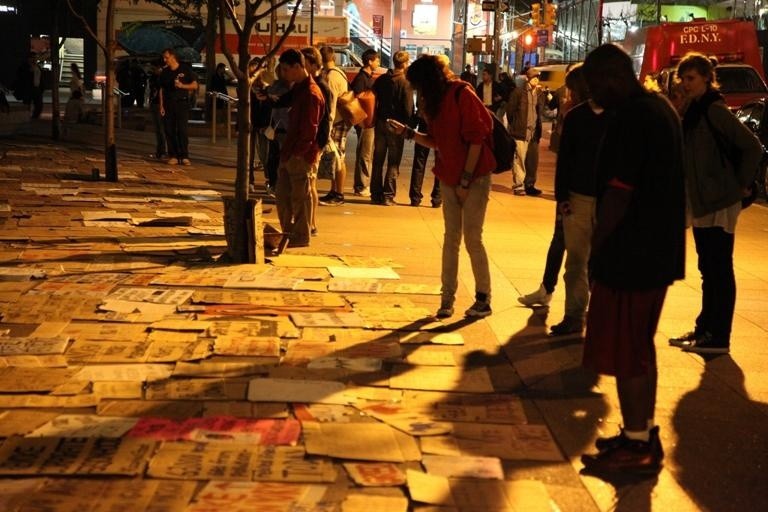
317, 151, 336, 180
336, 91, 368, 127
492, 111, 517, 175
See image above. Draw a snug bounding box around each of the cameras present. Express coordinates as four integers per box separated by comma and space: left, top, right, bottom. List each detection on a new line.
389, 122, 399, 130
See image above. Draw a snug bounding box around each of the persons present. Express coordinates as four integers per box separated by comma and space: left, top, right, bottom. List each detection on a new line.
516, 43, 768, 473
0, 51, 86, 143
115, 47, 199, 166
210, 45, 559, 318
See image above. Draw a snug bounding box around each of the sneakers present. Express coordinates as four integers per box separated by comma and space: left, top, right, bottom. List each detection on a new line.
181, 159, 192, 166
582, 444, 661, 477
437, 281, 731, 353
595, 426, 664, 459
317, 184, 444, 208
527, 188, 541, 195
514, 190, 526, 196
167, 158, 178, 165
